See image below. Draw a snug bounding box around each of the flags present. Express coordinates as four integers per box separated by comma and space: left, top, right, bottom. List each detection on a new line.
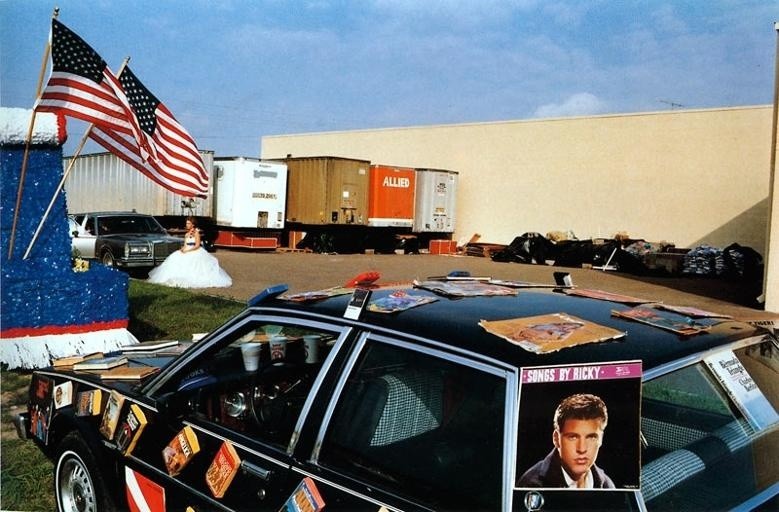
86, 65, 211, 199
33, 17, 163, 165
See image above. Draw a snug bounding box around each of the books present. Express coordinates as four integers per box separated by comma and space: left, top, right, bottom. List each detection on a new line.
205, 439, 241, 498
695, 363, 757, 437
115, 404, 148, 456
278, 476, 325, 512
705, 349, 779, 432
479, 311, 627, 356
53, 380, 73, 409
610, 304, 711, 336
161, 425, 200, 477
26, 374, 56, 446
98, 389, 126, 441
53, 332, 209, 382
660, 305, 733, 319
513, 360, 644, 492
73, 389, 102, 417
288, 274, 562, 316
564, 287, 663, 305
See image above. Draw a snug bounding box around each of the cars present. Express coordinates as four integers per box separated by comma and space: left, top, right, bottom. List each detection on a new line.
66, 211, 188, 271
13, 266, 779, 509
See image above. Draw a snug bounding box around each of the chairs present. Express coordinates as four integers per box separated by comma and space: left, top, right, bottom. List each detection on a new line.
336, 353, 756, 505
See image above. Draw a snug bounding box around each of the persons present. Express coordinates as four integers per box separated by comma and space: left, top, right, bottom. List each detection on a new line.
517, 393, 618, 488
178, 217, 201, 288
510, 320, 582, 349
